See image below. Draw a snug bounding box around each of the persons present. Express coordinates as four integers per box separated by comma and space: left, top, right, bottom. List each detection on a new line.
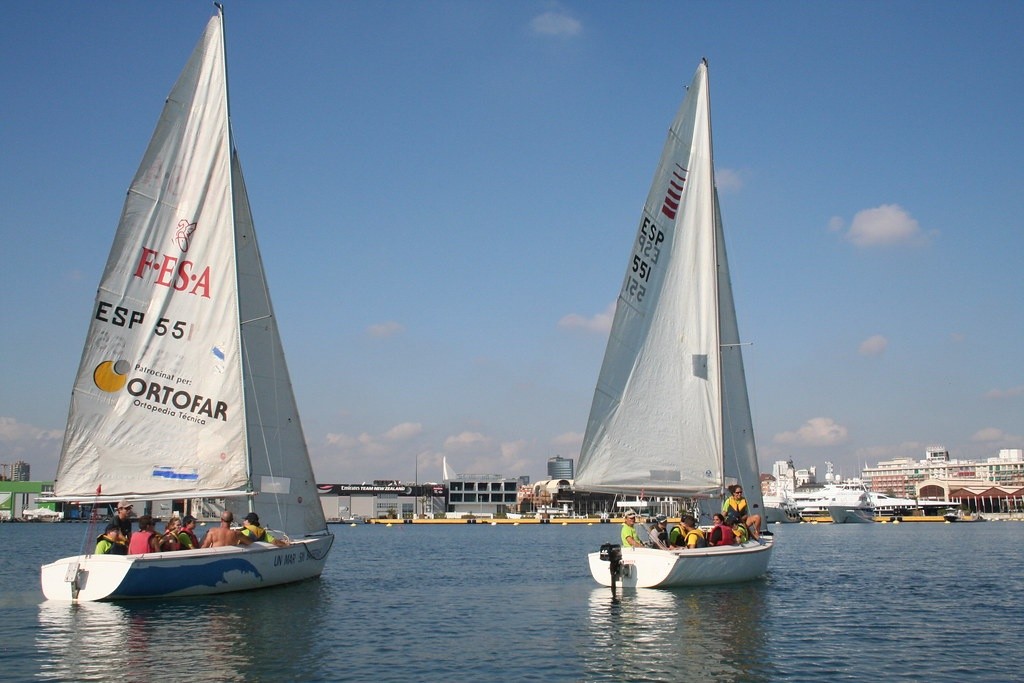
620, 510, 645, 547
707, 514, 734, 547
201, 511, 253, 549
238, 512, 289, 547
159, 517, 181, 551
649, 514, 670, 549
669, 515, 708, 549
95, 524, 122, 555
721, 485, 761, 545
104, 501, 134, 555
128, 515, 160, 555
178, 515, 200, 550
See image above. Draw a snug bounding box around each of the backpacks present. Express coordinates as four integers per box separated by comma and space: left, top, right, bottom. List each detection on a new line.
686, 533, 707, 549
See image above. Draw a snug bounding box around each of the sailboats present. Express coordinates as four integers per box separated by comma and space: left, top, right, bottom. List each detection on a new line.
568, 57, 773, 589
39, 0, 334, 603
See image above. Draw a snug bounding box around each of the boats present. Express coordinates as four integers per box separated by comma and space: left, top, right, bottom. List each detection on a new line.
813, 463, 875, 523
761, 474, 801, 522
943, 509, 987, 522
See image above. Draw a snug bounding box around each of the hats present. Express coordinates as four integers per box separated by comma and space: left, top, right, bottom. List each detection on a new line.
241, 512, 259, 521
138, 515, 162, 530
684, 516, 695, 527
183, 514, 198, 524
117, 500, 134, 508
624, 510, 638, 519
656, 513, 667, 524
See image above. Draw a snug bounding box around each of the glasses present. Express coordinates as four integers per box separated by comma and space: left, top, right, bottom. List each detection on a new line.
734, 491, 742, 494
